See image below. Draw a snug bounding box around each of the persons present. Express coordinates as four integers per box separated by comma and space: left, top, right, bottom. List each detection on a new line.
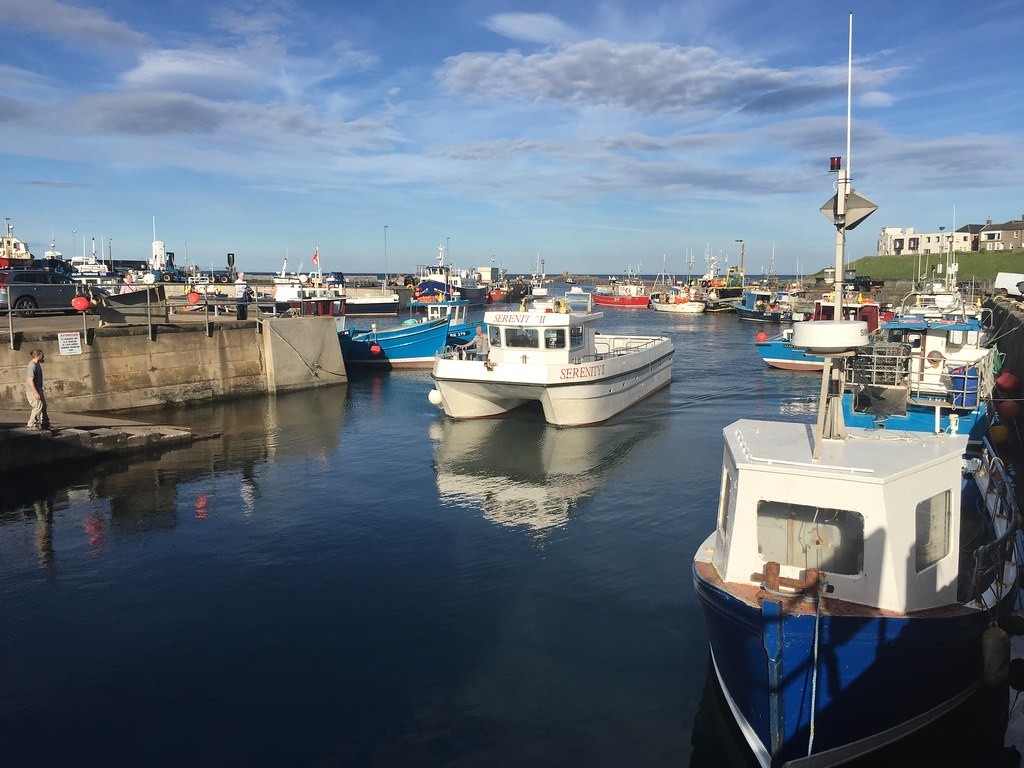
518, 275, 523, 284
234, 272, 248, 320
457, 326, 490, 361
54, 262, 64, 274
25, 349, 50, 431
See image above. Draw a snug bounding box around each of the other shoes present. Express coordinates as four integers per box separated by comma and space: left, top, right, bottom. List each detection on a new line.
27, 426, 41, 431
39, 424, 54, 430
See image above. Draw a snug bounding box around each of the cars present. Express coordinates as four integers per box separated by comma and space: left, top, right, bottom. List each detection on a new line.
0, 269, 109, 316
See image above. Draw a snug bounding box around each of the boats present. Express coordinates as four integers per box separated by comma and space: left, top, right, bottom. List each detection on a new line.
67, 236, 111, 276
753, 288, 897, 372
650, 240, 819, 323
428, 412, 665, 529
692, 200, 1024, 768
431, 297, 676, 426
277, 287, 488, 369
419, 237, 549, 304
591, 262, 648, 308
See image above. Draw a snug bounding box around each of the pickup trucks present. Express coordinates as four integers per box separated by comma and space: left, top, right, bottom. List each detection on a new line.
851, 276, 884, 293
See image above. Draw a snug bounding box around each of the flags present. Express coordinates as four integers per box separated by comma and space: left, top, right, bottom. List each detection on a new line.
312, 247, 319, 265
